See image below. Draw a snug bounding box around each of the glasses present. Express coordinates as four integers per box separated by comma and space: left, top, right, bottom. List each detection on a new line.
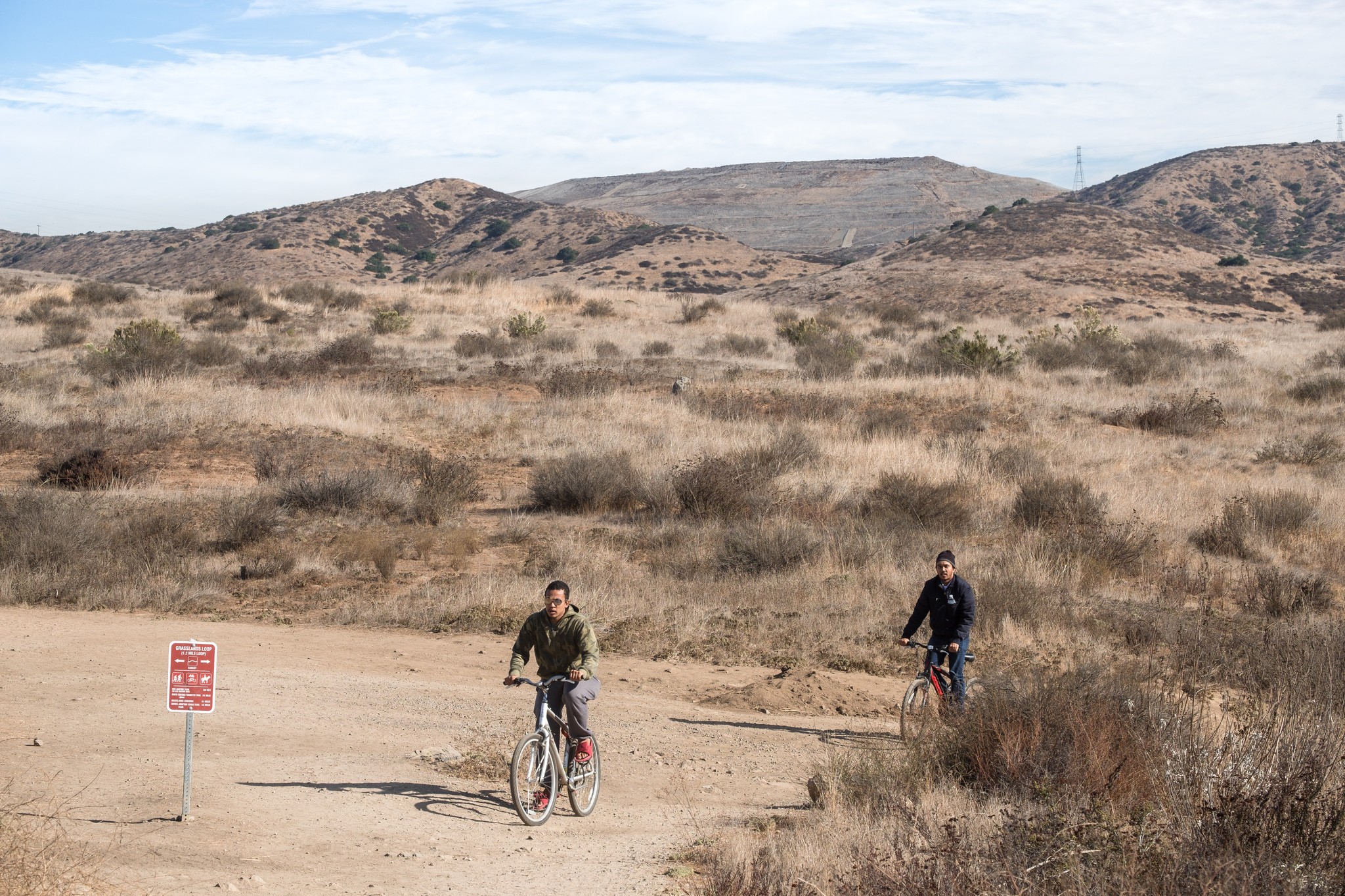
544, 596, 568, 605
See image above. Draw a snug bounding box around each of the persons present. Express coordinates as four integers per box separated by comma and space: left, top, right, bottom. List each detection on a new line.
896, 550, 975, 725
504, 581, 600, 811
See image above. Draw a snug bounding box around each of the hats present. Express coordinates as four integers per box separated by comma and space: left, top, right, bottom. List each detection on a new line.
936, 550, 956, 569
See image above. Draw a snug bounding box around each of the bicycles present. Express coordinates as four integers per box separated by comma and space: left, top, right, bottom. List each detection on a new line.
502, 670, 601, 827
901, 639, 987, 751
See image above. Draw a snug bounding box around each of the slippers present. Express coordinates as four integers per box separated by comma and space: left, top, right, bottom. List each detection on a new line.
532, 790, 559, 809
574, 741, 593, 762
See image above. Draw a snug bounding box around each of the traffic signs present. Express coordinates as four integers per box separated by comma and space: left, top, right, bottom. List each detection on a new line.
167, 641, 218, 714
